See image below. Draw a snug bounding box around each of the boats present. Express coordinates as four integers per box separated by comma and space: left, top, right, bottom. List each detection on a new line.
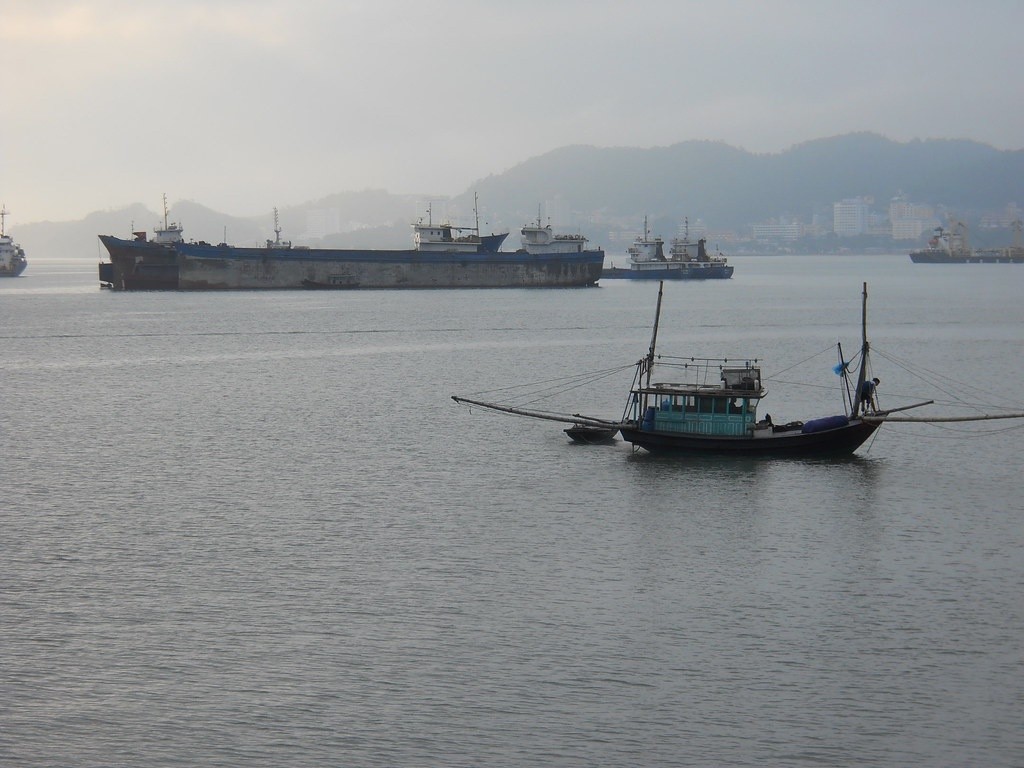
563, 412, 619, 444
0, 205, 28, 277
620, 278, 887, 458
98, 190, 603, 291
601, 215, 734, 281
907, 223, 1024, 263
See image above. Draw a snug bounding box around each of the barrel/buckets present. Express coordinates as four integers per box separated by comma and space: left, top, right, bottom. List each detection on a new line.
642, 406, 660, 432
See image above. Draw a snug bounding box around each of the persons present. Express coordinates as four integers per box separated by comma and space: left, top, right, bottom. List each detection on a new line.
859, 378, 880, 414
729, 396, 743, 414
